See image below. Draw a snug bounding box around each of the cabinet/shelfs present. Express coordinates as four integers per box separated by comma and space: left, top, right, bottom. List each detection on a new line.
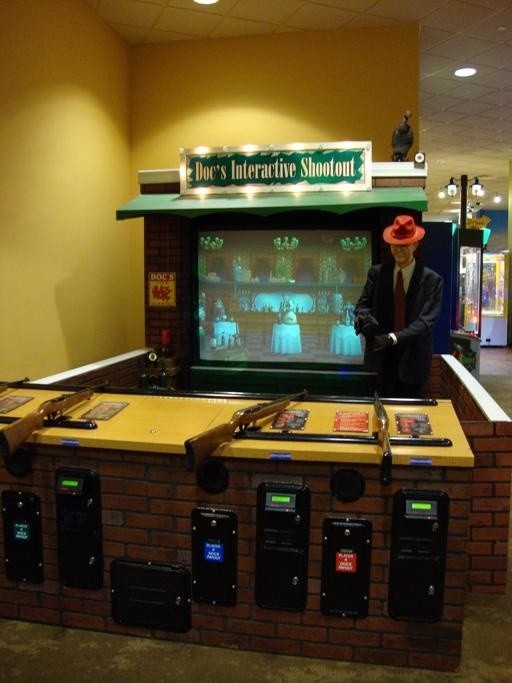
199, 270, 366, 335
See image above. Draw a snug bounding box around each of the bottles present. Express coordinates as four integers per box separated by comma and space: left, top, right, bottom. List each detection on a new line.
158, 368, 167, 387
335, 310, 354, 326
209, 312, 243, 350
251, 298, 316, 324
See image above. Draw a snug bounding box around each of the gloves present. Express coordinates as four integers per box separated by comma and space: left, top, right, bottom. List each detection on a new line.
354, 315, 379, 335
370, 334, 392, 352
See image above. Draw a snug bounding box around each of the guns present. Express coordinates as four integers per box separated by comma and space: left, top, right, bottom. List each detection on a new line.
1, 379, 113, 463
1, 377, 31, 393
374, 393, 391, 486
184, 389, 309, 472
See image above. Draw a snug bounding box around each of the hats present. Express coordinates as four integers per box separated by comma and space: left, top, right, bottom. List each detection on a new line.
383, 215, 425, 245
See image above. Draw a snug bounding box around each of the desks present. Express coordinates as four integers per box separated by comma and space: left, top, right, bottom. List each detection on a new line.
269, 322, 301, 355
208, 319, 237, 345
329, 323, 362, 357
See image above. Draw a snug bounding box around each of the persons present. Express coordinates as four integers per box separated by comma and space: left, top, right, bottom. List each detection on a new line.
355, 214, 442, 398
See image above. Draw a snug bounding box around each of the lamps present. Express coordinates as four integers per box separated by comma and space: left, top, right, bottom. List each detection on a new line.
444, 175, 485, 230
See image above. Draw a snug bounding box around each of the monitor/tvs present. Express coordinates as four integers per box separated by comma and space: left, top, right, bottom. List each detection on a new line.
185, 222, 378, 374
